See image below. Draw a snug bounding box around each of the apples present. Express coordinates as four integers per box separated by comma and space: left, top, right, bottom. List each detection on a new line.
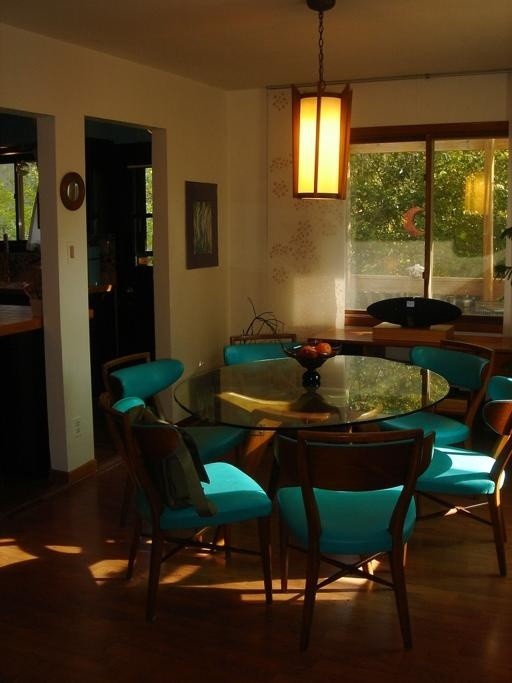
299, 345, 316, 355
316, 343, 332, 353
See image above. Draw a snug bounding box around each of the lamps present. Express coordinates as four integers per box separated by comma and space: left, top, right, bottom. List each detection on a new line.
465, 172, 484, 215
292, 0, 353, 199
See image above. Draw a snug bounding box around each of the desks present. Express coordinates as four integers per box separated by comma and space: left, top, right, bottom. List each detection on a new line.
309, 324, 512, 376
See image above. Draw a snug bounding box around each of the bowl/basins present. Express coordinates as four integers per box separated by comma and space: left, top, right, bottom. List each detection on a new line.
288, 383, 336, 412
282, 342, 343, 384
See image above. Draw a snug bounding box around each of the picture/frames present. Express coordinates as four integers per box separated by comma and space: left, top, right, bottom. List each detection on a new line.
185, 180, 219, 269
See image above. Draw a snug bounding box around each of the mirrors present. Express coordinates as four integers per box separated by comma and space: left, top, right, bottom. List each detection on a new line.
60, 170, 86, 210
